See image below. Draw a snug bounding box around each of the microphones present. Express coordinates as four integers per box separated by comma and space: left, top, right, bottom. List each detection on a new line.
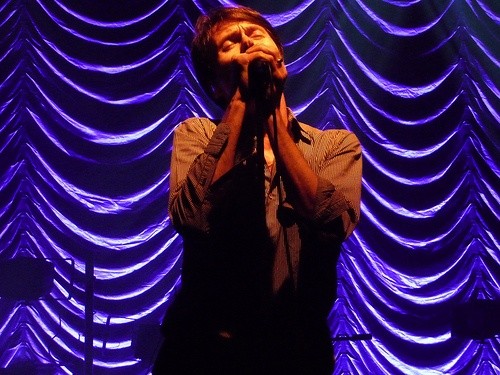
250, 58, 277, 102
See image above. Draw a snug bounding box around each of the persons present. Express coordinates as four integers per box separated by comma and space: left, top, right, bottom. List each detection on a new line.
151, 7, 363, 375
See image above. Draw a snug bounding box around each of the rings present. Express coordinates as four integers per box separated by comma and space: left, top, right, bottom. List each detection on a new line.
276, 57, 283, 67
